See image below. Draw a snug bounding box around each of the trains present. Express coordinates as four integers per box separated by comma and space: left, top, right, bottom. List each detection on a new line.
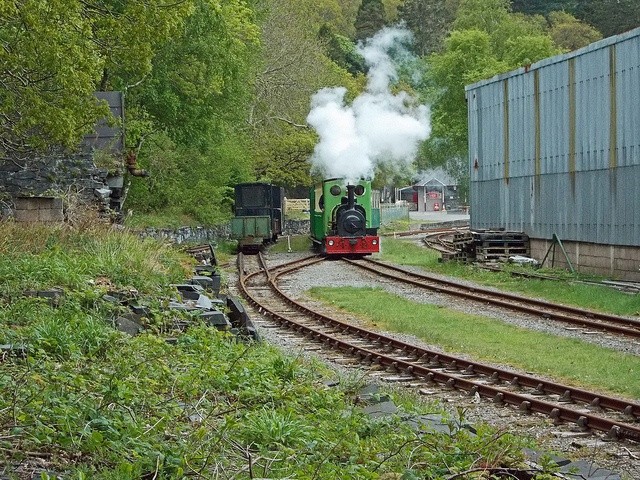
231, 183, 287, 255
308, 176, 380, 257
